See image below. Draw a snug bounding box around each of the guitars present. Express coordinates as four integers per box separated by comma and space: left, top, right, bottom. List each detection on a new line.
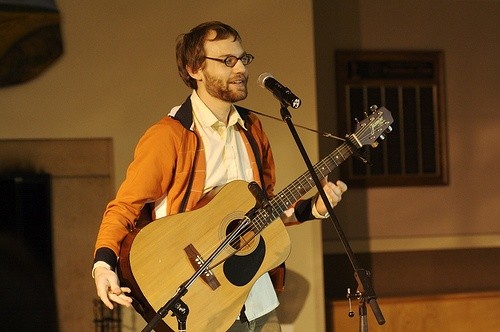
117, 104, 394, 332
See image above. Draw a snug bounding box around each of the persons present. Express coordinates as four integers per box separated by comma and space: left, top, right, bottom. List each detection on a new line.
91, 22, 347, 332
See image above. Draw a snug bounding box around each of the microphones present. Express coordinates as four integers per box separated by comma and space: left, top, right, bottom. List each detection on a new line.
248, 181, 273, 214
257, 72, 301, 109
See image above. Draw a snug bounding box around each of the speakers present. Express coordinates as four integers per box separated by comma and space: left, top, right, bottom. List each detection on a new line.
0, 166, 60, 332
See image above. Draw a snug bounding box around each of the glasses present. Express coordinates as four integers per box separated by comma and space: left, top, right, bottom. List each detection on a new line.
204, 53, 255, 68
